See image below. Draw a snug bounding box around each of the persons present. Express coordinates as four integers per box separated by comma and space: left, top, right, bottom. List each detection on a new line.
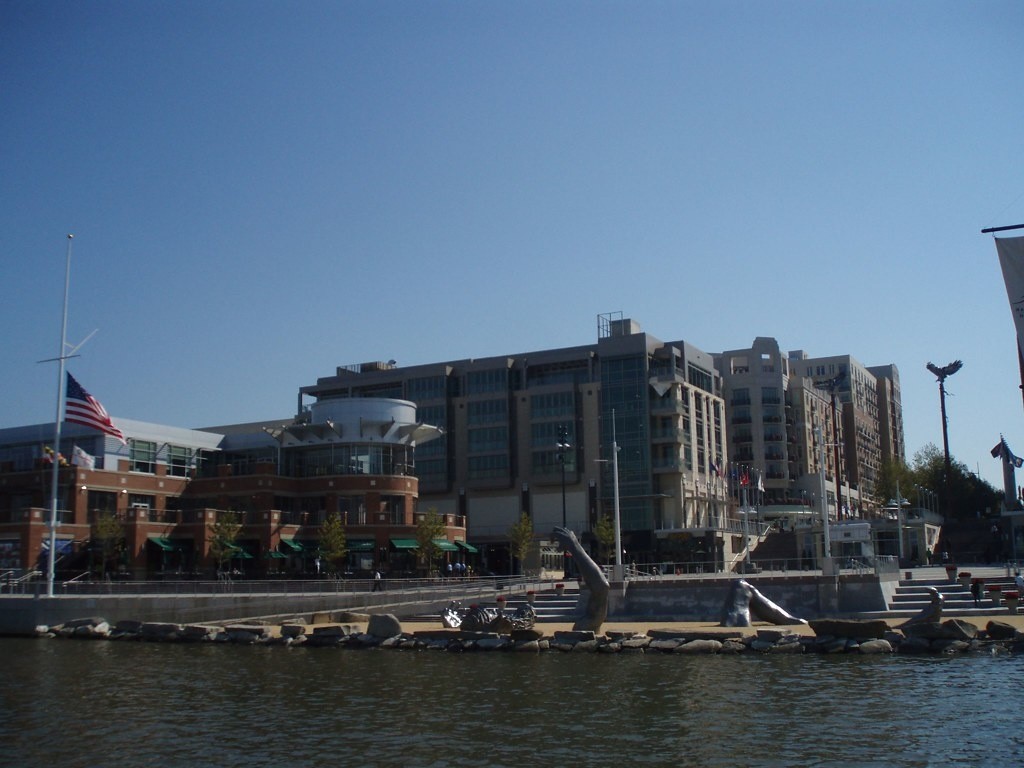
371, 570, 382, 593
926, 548, 936, 567
315, 557, 322, 575
942, 550, 948, 564
844, 503, 864, 518
447, 560, 473, 578
1014, 573, 1024, 598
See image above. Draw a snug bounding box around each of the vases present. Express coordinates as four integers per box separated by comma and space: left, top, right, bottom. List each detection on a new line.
976, 584, 983, 595
947, 570, 957, 581
527, 594, 536, 602
1006, 599, 1018, 614
960, 577, 969, 586
497, 601, 506, 609
990, 590, 1001, 605
556, 588, 564, 595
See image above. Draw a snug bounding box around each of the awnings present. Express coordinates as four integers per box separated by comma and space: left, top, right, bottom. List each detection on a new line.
455, 542, 478, 553
431, 540, 460, 553
230, 543, 260, 558
281, 539, 303, 552
148, 537, 182, 554
264, 551, 288, 559
348, 541, 375, 552
390, 540, 422, 549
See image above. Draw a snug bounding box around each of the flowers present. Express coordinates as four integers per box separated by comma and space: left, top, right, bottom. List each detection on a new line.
986, 585, 1000, 590
946, 566, 957, 570
1004, 592, 1020, 599
972, 578, 984, 583
496, 596, 505, 601
527, 591, 534, 595
556, 584, 564, 588
958, 572, 970, 577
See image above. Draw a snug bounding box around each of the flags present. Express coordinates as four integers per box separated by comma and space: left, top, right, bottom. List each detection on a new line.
713, 460, 765, 492
65, 370, 125, 442
44, 447, 95, 471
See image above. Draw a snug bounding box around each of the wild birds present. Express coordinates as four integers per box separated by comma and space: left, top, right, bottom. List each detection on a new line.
927, 360, 963, 384
